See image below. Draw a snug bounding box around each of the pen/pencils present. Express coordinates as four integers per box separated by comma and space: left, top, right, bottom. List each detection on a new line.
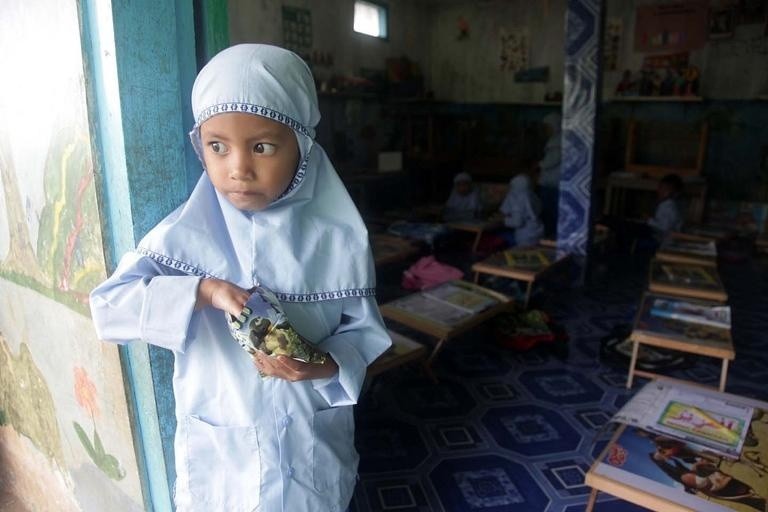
691, 406, 739, 441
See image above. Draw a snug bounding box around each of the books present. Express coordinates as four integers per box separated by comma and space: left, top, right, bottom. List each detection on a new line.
367, 245, 564, 371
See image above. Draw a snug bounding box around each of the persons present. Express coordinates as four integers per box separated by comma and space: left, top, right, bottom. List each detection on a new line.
444, 171, 483, 220
88, 43, 393, 512
528, 109, 562, 222
636, 174, 685, 269
494, 172, 546, 249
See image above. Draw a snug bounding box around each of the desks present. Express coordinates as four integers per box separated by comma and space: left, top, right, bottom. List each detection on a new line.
628, 292, 735, 393
585, 380, 768, 512
372, 201, 577, 385
648, 226, 728, 300
